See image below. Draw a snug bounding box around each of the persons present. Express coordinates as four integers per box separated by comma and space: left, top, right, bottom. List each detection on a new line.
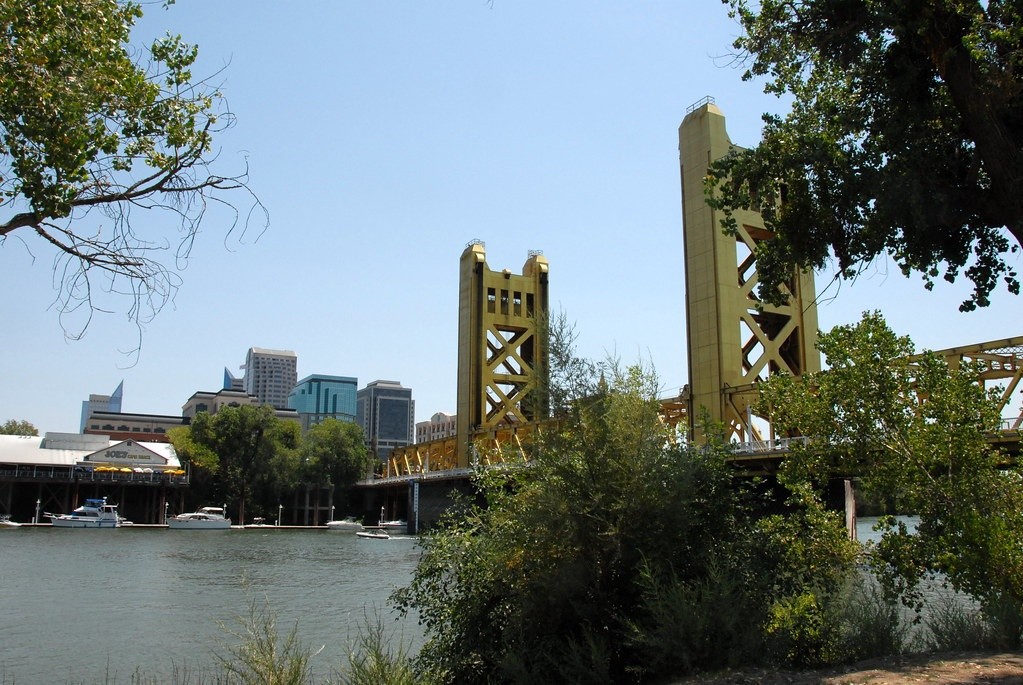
369, 530, 377, 535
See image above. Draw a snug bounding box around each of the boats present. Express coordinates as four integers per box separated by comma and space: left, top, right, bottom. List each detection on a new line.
381, 519, 408, 530
327, 517, 365, 531
0, 514, 21, 530
166, 506, 232, 529
43, 497, 121, 528
356, 528, 389, 539
